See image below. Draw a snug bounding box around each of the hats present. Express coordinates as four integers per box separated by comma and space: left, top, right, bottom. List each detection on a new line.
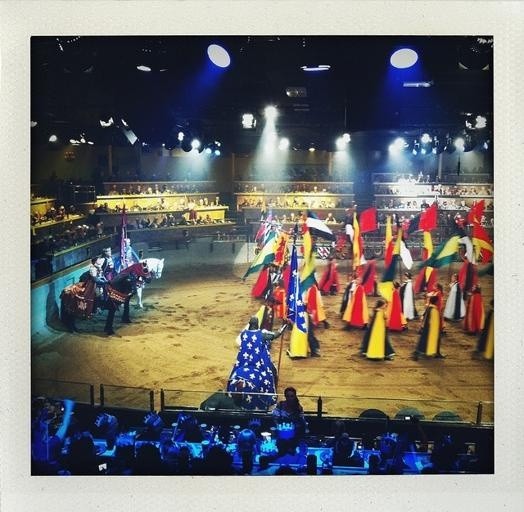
404, 272, 412, 279
91, 257, 99, 264
103, 246, 112, 252
124, 238, 131, 243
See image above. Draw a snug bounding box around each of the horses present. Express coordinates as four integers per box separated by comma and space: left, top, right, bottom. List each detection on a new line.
115, 258, 165, 308
80, 260, 153, 323
60, 272, 137, 335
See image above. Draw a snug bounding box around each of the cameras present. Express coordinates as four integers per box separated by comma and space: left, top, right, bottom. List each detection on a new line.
404, 415, 411, 421
98, 462, 108, 472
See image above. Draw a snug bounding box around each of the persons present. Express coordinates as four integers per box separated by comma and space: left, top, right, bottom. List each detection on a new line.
101, 245, 119, 279
234, 168, 494, 228
122, 238, 139, 269
240, 199, 493, 362
89, 252, 107, 316
227, 315, 287, 412
30, 171, 230, 260
32, 387, 483, 477
